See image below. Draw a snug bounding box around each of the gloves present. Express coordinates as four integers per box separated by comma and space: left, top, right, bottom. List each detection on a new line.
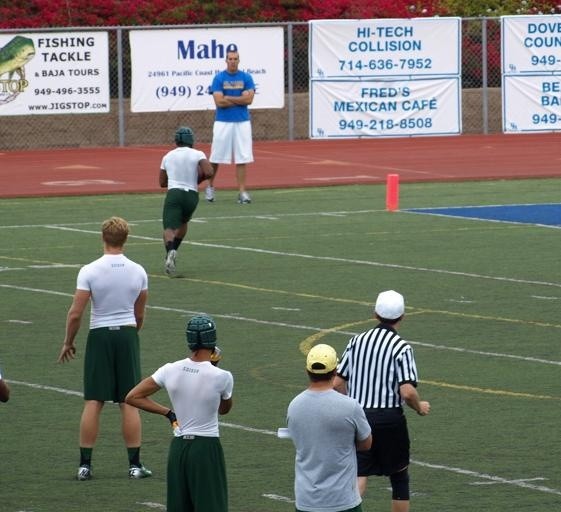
164, 409, 183, 438
209, 345, 223, 367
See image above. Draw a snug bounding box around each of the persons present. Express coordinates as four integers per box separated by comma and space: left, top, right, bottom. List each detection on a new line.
59, 215, 152, 480
158, 123, 213, 275
332, 289, 431, 510
204, 49, 256, 203
284, 344, 372, 512
125, 314, 234, 511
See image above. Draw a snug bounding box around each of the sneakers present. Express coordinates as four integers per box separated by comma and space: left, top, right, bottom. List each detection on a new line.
127, 463, 154, 480
236, 190, 253, 206
204, 185, 217, 202
75, 465, 98, 482
163, 248, 179, 278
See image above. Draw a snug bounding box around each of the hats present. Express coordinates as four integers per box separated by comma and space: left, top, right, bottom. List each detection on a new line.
306, 343, 341, 374
373, 288, 407, 321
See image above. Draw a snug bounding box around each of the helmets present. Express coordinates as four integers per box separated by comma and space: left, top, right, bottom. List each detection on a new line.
174, 126, 197, 147
185, 315, 218, 352
